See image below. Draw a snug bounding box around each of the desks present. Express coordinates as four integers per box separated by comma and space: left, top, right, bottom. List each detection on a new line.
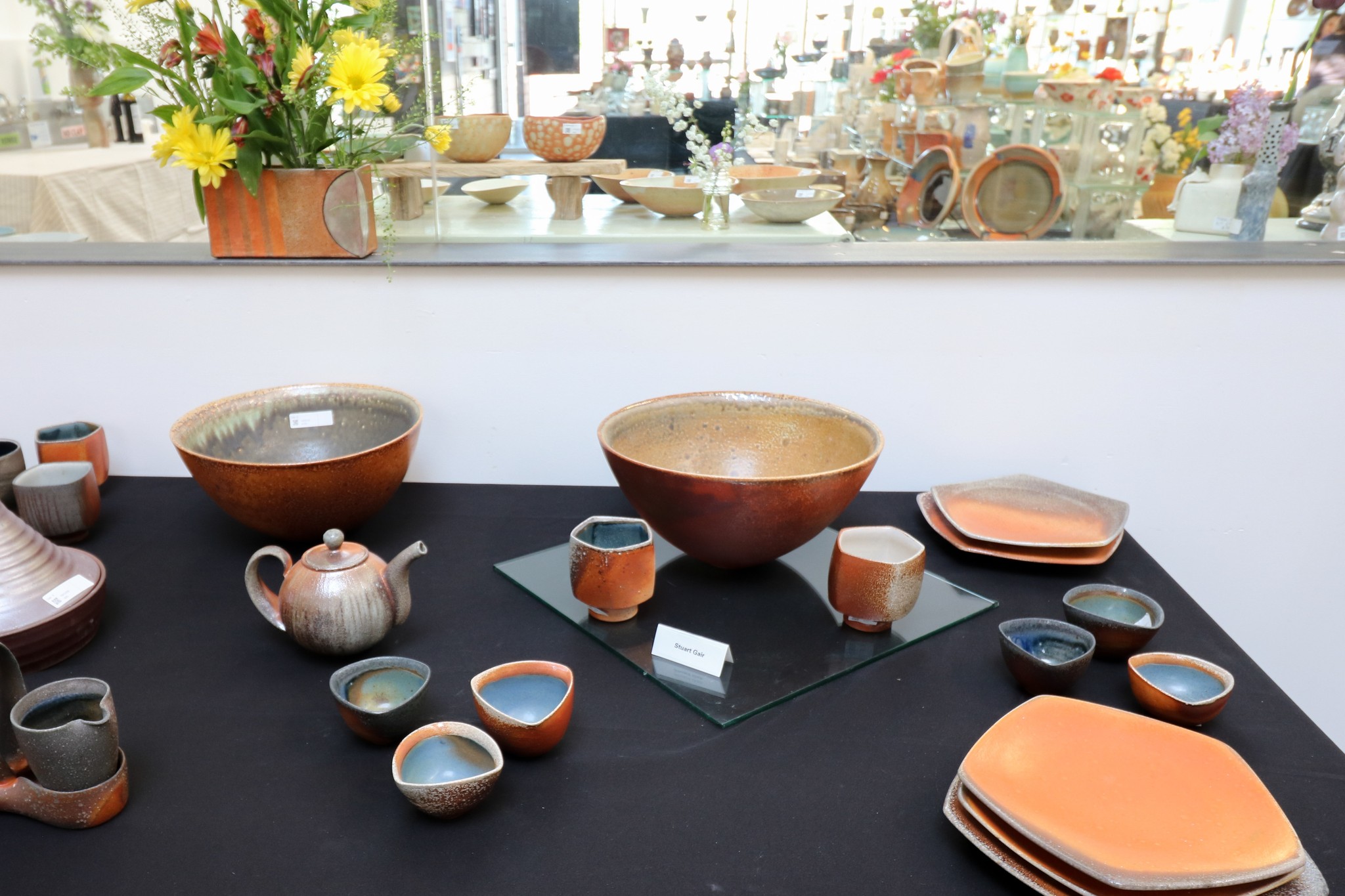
0, 477, 1345, 896
0, 138, 198, 244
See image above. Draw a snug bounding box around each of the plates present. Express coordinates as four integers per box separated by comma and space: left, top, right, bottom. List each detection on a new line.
943, 693, 1332, 896
915, 473, 1130, 565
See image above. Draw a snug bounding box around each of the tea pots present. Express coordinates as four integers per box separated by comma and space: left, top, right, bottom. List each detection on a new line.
244, 529, 429, 658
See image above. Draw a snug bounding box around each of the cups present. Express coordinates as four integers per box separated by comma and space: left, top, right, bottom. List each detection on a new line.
329, 655, 575, 815
570, 515, 654, 624
999, 583, 1236, 730
827, 525, 926, 632
893, 51, 1043, 108
0, 421, 109, 547
9, 675, 119, 791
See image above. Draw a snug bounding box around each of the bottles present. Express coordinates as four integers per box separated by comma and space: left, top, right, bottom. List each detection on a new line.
110, 91, 145, 143
836, 151, 894, 229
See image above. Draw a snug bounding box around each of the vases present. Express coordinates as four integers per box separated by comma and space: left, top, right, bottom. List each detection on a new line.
201, 163, 378, 258
1209, 165, 1244, 214
1143, 174, 1185, 216
702, 182, 736, 228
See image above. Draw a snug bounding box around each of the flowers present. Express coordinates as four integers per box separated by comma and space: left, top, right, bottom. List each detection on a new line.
647, 77, 757, 178
1153, 86, 1289, 165
125, 4, 445, 172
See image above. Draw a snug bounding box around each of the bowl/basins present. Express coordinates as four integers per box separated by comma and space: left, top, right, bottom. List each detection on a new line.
545, 178, 591, 204
524, 115, 606, 162
169, 382, 422, 542
593, 164, 846, 223
434, 115, 511, 163
460, 179, 530, 204
597, 391, 882, 566
896, 145, 1065, 241
419, 179, 453, 204
1043, 82, 1161, 114
